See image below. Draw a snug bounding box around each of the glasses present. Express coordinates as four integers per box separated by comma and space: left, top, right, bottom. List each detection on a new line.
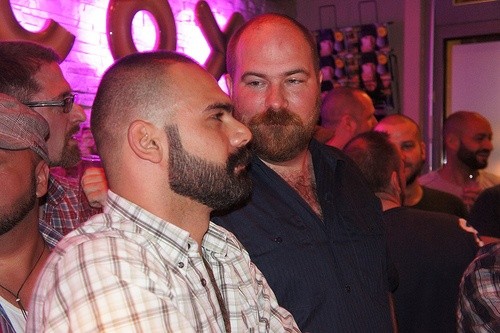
23, 93, 80, 113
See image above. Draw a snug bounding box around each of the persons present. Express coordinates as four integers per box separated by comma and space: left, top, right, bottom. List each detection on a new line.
0, 13, 500, 333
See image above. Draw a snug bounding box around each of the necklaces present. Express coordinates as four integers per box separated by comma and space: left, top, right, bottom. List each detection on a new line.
0, 233, 45, 320
377, 194, 400, 206
273, 150, 322, 211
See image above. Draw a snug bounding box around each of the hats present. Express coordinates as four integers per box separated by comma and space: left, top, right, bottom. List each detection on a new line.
0, 93, 51, 163
